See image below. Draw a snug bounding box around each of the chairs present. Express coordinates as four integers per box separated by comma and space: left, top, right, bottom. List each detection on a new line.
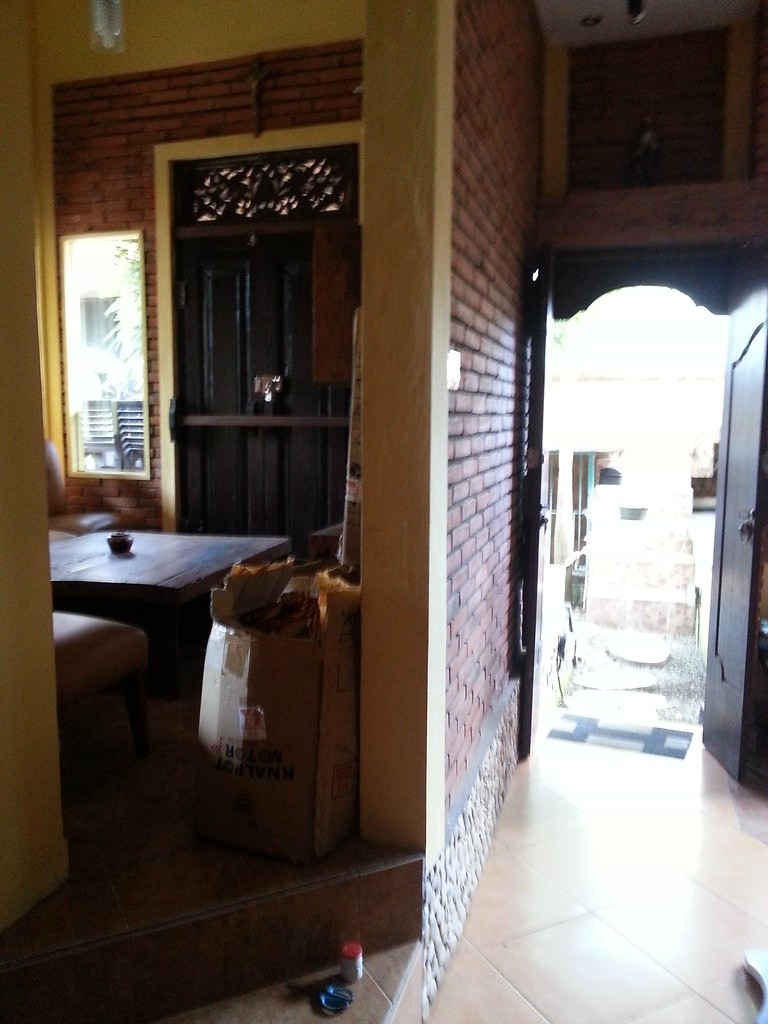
44, 437, 149, 536
52, 610, 150, 758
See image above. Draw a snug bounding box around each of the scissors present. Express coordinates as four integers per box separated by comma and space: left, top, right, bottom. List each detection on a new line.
284, 983, 353, 1012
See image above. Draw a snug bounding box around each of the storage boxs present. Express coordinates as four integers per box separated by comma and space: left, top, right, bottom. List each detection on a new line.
193, 553, 361, 868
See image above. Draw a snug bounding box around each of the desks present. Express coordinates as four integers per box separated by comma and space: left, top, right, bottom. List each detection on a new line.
49, 531, 293, 703
